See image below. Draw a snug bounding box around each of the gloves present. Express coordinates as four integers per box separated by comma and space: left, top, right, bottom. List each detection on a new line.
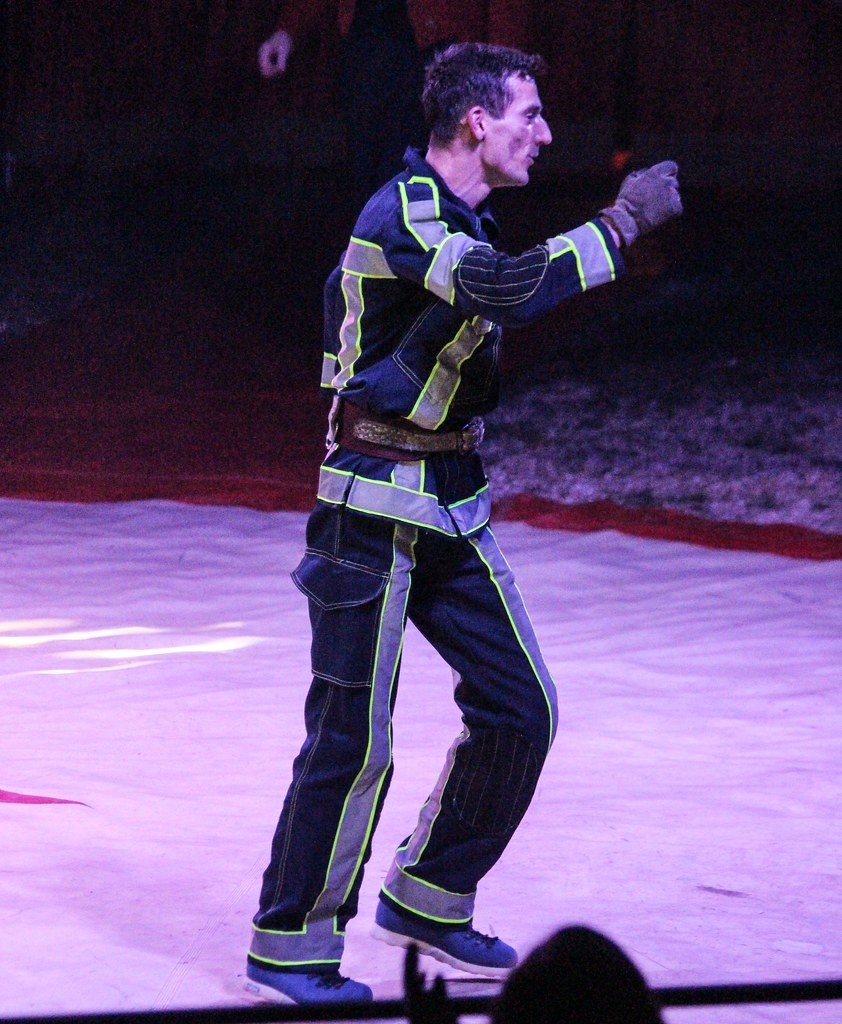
598, 159, 686, 249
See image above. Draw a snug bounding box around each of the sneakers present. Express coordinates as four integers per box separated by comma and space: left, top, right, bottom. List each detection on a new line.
243, 962, 373, 1007
370, 898, 519, 977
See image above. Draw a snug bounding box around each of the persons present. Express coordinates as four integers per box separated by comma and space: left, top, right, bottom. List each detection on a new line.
243, 38, 685, 1008
401, 925, 676, 1024
